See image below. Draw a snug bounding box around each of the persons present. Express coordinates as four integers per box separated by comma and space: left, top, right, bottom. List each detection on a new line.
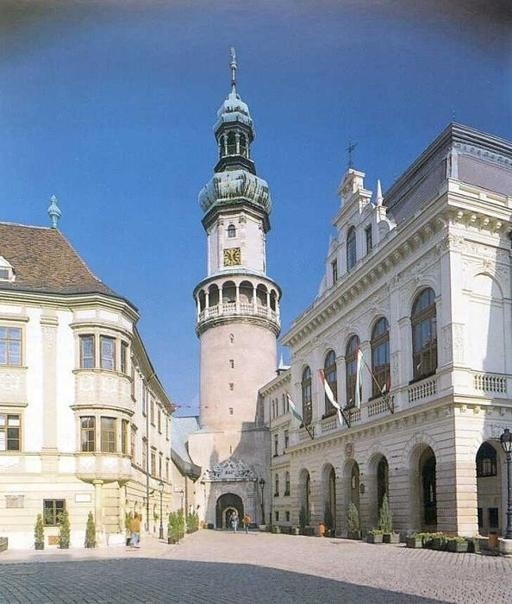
229, 511, 240, 532
130, 514, 141, 548
241, 514, 252, 534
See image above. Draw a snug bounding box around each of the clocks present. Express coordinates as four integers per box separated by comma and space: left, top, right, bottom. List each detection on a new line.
224, 247, 241, 266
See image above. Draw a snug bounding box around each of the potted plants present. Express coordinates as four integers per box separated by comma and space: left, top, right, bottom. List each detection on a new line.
448, 537, 470, 553
57, 509, 71, 549
177, 513, 184, 539
185, 511, 201, 534
168, 512, 179, 544
257, 501, 335, 536
378, 494, 400, 544
426, 534, 447, 550
406, 532, 423, 548
345, 502, 363, 541
125, 512, 142, 546
366, 529, 383, 542
84, 511, 97, 549
476, 537, 502, 556
34, 512, 45, 550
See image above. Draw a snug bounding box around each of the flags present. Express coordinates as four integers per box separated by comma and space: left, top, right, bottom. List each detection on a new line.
354, 349, 367, 409
319, 368, 343, 427
287, 394, 303, 432
